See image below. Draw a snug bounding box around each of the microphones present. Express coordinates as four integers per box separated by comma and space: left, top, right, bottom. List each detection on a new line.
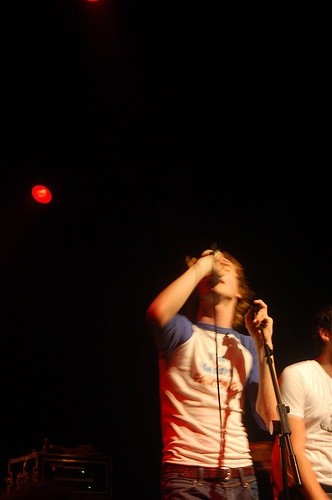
209, 252, 219, 284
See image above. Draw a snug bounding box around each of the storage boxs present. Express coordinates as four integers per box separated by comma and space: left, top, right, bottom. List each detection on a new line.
7, 452, 114, 500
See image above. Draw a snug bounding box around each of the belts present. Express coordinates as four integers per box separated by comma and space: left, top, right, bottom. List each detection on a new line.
320, 483, 332, 493
162, 463, 255, 481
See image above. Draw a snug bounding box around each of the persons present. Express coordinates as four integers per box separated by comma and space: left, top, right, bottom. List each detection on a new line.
278, 307, 332, 500
145, 249, 283, 500
